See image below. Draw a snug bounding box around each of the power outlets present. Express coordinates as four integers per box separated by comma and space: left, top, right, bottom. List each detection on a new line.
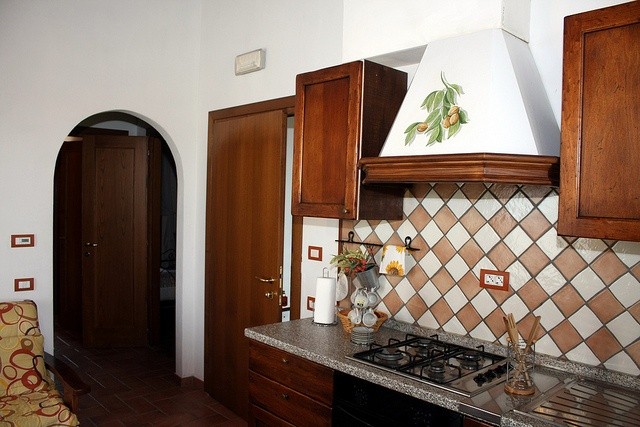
480, 269, 510, 292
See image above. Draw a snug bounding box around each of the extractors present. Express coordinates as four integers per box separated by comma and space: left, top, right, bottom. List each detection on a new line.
340, 3, 561, 181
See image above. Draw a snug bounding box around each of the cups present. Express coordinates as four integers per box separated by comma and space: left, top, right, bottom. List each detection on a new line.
364, 312, 380, 328
350, 285, 381, 308
347, 311, 360, 327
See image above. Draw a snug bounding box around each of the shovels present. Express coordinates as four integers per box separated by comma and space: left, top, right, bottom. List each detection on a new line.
503, 316, 511, 340
511, 316, 540, 389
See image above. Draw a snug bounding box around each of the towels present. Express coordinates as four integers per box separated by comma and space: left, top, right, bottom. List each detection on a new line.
379, 245, 410, 276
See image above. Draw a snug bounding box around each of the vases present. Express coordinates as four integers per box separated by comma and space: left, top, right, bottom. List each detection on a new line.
355, 264, 380, 291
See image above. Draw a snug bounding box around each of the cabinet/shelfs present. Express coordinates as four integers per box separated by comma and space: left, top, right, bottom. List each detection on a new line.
245, 328, 334, 427
291, 58, 404, 222
558, 0, 637, 242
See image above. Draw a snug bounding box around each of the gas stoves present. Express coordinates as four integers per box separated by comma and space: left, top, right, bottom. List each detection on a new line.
347, 333, 536, 397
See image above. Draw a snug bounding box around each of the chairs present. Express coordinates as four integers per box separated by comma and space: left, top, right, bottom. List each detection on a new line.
0, 300, 79, 427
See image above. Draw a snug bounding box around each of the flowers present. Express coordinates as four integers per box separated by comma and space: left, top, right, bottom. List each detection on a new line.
330, 245, 374, 276
